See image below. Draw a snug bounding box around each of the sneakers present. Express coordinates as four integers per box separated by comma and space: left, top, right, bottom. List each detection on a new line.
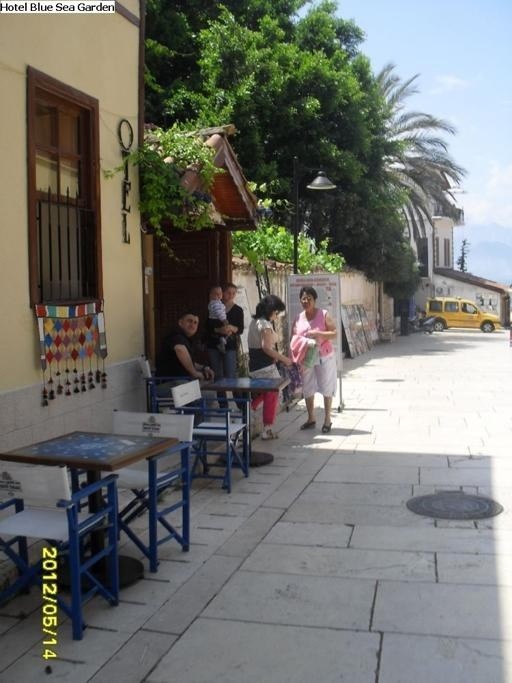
262, 429, 278, 439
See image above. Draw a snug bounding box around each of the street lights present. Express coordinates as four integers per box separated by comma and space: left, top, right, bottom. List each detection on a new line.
291, 156, 338, 274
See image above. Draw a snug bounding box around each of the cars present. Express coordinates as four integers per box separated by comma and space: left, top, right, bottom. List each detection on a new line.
428, 298, 501, 332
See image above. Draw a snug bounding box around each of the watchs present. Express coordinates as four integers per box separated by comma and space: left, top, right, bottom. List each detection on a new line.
201, 366, 206, 371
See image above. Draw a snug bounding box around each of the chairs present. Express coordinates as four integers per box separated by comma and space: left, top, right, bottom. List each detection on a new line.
170, 378, 252, 495
102, 407, 194, 574
0, 473, 119, 642
137, 353, 194, 414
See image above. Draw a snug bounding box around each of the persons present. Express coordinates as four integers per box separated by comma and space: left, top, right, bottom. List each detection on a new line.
460, 300, 468, 310
210, 283, 247, 413
290, 287, 337, 433
248, 296, 292, 440
208, 285, 229, 352
155, 311, 215, 398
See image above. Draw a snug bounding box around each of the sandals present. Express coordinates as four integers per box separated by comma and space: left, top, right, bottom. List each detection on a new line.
301, 420, 315, 430
322, 422, 332, 433
